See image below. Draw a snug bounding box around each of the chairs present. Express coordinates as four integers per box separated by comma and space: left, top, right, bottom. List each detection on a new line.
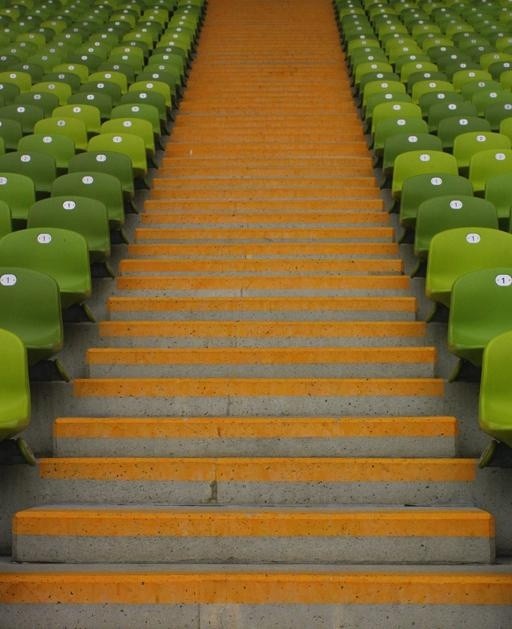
1, 0, 208, 468
333, 1, 510, 467
